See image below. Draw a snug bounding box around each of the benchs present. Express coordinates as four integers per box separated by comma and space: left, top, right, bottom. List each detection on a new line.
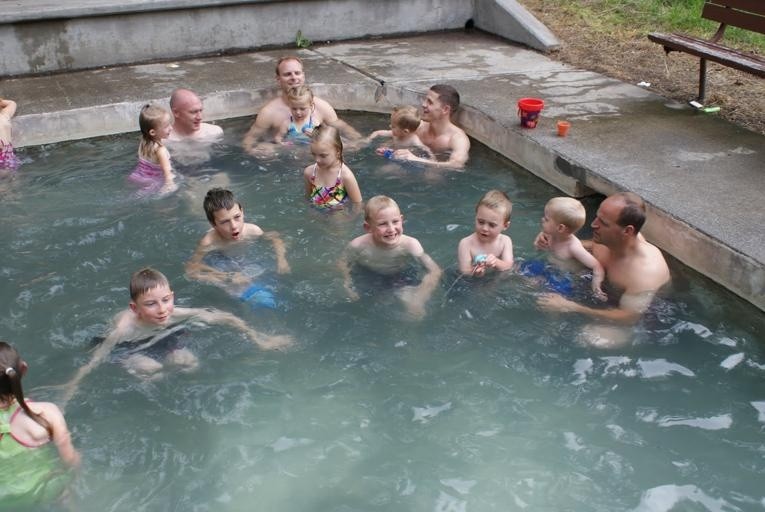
647, 0, 765, 99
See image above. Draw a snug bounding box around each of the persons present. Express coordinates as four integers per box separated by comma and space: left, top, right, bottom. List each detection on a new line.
0, 98, 18, 194
335, 193, 443, 323
456, 188, 674, 349
302, 121, 363, 237
51, 266, 294, 414
241, 55, 471, 185
163, 88, 232, 220
0, 339, 84, 512
182, 185, 291, 311
123, 98, 180, 203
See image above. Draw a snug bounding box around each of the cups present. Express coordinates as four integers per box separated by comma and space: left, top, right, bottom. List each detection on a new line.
558, 121, 570, 136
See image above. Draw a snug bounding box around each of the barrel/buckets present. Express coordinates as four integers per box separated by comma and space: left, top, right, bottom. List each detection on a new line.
517, 98, 544, 128
